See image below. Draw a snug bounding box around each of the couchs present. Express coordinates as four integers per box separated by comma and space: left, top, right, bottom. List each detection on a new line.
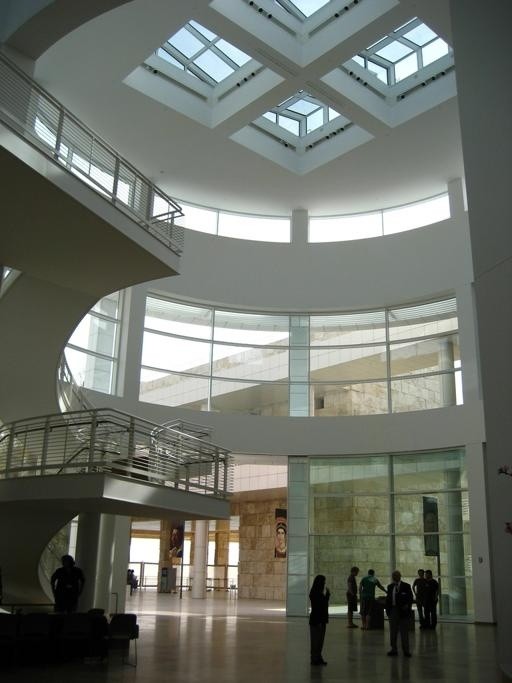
0, 613, 110, 669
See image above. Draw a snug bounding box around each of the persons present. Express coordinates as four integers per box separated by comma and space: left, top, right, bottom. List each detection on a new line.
50, 554, 86, 611
128, 569, 138, 589
170, 527, 183, 558
384, 570, 414, 658
412, 568, 428, 629
346, 566, 360, 629
274, 522, 287, 558
307, 574, 332, 667
358, 569, 388, 631
420, 568, 440, 631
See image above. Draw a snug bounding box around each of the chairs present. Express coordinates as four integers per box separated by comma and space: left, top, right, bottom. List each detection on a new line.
0, 615, 92, 668
110, 614, 138, 668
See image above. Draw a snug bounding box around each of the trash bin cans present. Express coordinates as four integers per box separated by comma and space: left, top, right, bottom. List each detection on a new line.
362, 598, 384, 630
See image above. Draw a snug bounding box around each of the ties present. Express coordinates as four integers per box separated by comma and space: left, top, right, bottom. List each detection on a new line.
394, 585, 399, 605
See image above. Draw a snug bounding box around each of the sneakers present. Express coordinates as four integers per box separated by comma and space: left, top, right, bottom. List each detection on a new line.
387, 649, 412, 657
345, 620, 377, 631
310, 658, 327, 665
419, 622, 438, 631
